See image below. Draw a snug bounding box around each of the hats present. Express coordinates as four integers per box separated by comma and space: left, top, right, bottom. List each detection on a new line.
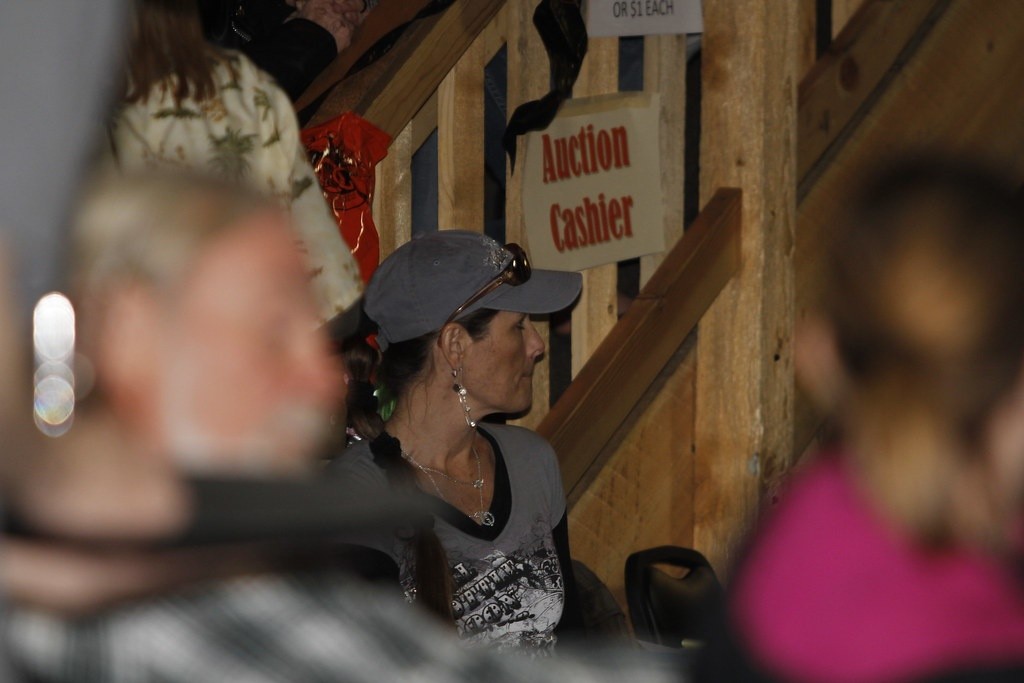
362, 230, 582, 353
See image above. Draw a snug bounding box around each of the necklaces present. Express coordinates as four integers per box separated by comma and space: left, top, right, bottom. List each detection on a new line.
401, 447, 495, 526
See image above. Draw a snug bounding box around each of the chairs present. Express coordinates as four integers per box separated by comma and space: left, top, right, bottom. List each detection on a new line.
623, 543, 724, 648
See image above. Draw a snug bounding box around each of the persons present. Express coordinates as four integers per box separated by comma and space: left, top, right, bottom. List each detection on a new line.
324, 227, 584, 661
104, 0, 366, 339
0, 161, 519, 683
734, 161, 1024, 683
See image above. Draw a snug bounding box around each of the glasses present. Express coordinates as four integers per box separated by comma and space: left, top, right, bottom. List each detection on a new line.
437, 243, 532, 348
